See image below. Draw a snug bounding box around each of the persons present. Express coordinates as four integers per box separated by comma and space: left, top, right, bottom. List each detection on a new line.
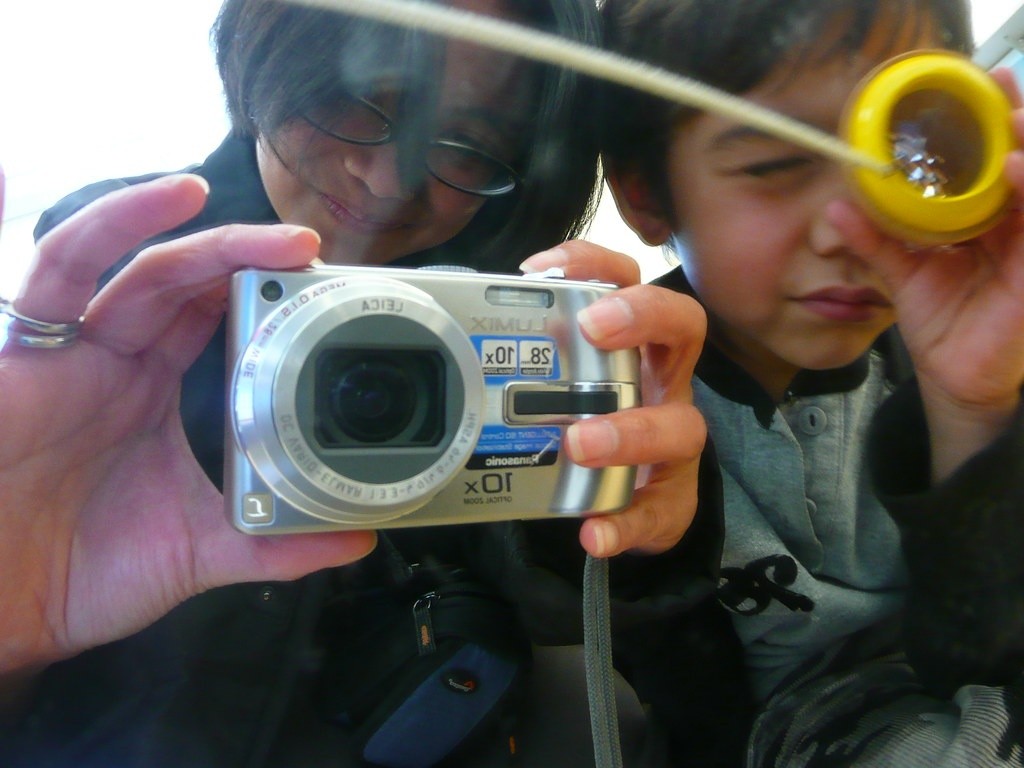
0, 0, 746, 767
585, 1, 1024, 767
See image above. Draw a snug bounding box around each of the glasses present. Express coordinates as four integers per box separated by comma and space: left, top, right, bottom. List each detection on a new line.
296, 86, 524, 200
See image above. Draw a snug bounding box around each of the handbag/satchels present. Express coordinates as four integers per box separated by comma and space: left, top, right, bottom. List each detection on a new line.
263, 561, 538, 768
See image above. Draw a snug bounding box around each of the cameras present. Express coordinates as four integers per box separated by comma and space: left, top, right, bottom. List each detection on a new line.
224, 255, 642, 536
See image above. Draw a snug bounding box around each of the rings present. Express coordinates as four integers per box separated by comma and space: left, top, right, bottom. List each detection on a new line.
0, 298, 83, 333
3, 322, 80, 347
